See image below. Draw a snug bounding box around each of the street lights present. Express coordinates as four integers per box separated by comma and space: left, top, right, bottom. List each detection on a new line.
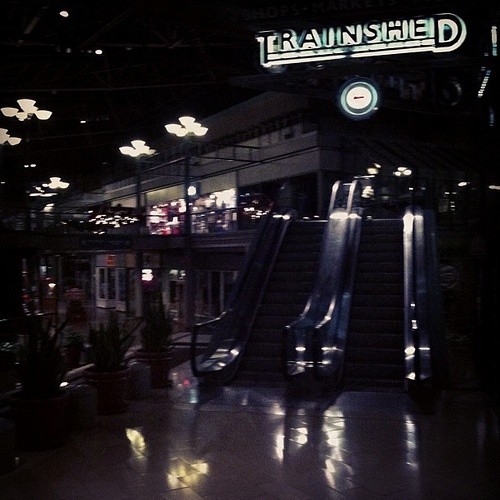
119, 139, 158, 327
109, 215, 139, 318
164, 116, 208, 331
1, 99, 52, 367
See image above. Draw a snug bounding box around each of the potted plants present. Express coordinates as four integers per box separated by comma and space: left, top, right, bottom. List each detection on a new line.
9, 315, 72, 448
85, 321, 135, 410
135, 316, 176, 390
